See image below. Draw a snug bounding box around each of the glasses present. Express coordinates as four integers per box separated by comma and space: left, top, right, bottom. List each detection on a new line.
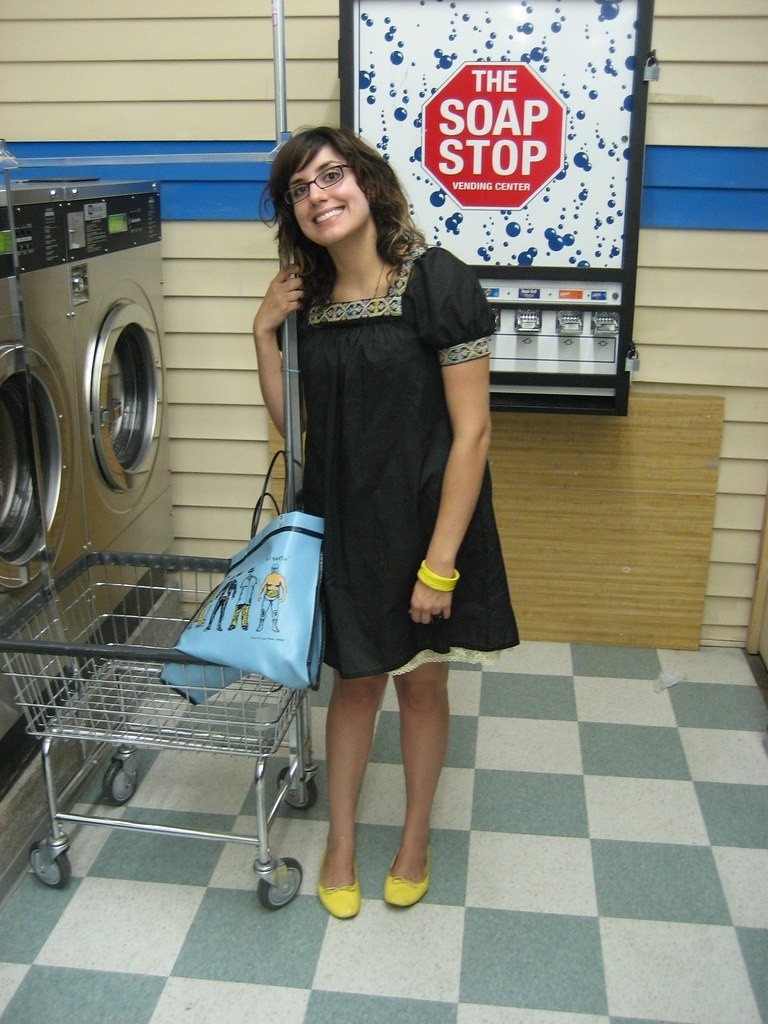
284, 164, 352, 205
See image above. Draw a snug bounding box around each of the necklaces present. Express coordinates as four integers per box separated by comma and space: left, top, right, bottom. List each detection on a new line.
331, 261, 386, 303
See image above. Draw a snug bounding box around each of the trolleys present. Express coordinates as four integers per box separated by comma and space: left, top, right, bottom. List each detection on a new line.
1, 550, 321, 911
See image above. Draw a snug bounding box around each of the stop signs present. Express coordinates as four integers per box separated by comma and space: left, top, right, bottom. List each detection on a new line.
421, 62, 566, 211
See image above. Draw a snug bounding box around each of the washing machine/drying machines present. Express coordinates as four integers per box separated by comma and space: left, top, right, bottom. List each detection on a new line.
0, 177, 171, 796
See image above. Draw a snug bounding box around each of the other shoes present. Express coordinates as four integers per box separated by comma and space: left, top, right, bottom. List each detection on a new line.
318, 846, 361, 920
382, 839, 431, 907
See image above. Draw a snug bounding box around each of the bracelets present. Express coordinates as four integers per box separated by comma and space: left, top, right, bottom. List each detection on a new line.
417, 562, 460, 591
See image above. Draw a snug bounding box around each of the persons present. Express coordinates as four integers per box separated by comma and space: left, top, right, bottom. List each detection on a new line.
253, 125, 519, 919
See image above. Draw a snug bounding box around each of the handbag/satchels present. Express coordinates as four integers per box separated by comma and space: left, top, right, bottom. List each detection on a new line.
158, 450, 328, 707
